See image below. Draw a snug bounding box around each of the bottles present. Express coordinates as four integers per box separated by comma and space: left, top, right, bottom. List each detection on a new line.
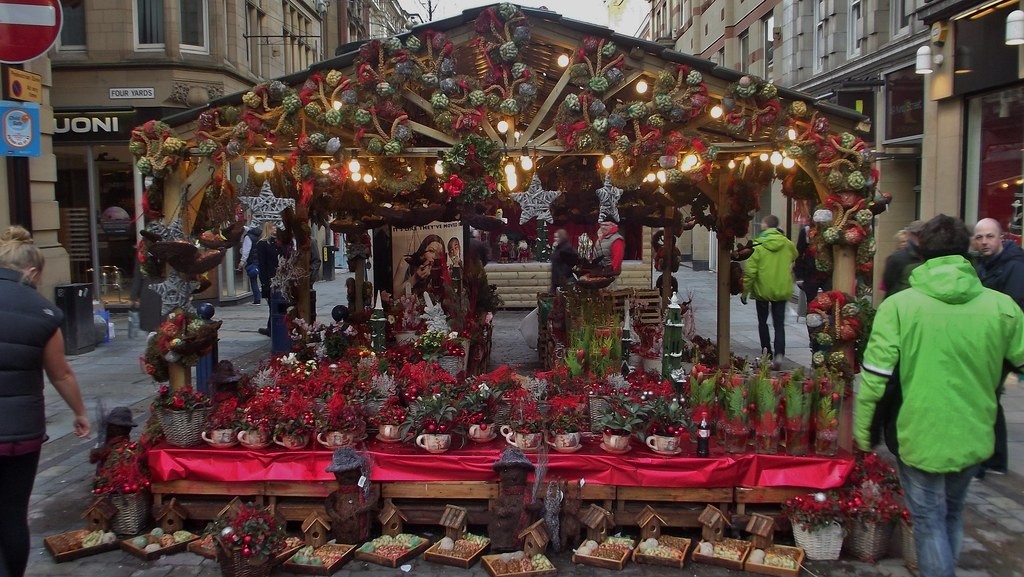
697, 412, 711, 457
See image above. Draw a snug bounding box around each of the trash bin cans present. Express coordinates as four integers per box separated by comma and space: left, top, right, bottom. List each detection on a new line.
57, 285, 97, 355
269, 290, 317, 354
323, 245, 336, 281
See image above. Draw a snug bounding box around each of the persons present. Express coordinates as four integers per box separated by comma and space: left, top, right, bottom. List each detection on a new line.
130, 260, 162, 334
854, 213, 1024, 577
393, 235, 462, 301
0, 225, 92, 577
551, 213, 626, 298
741, 215, 833, 373
238, 220, 321, 337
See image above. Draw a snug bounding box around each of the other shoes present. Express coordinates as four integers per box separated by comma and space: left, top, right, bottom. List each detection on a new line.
985, 465, 1007, 476
258, 328, 271, 337
763, 347, 783, 371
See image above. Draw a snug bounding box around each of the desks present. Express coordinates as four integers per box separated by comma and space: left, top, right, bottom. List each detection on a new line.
143, 436, 856, 530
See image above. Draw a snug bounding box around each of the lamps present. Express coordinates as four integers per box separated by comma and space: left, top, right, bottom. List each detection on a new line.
915, 45, 943, 74
954, 46, 977, 73
1004, 9, 1024, 45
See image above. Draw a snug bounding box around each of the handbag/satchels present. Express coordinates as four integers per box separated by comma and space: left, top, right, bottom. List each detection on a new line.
246, 265, 260, 278
